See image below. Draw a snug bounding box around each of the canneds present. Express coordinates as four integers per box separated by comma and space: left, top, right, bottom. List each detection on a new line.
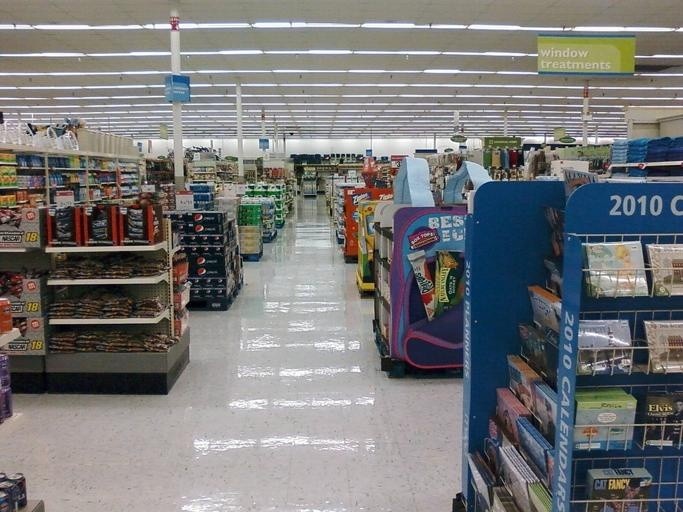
0, 354, 13, 424
0, 472, 27, 512
